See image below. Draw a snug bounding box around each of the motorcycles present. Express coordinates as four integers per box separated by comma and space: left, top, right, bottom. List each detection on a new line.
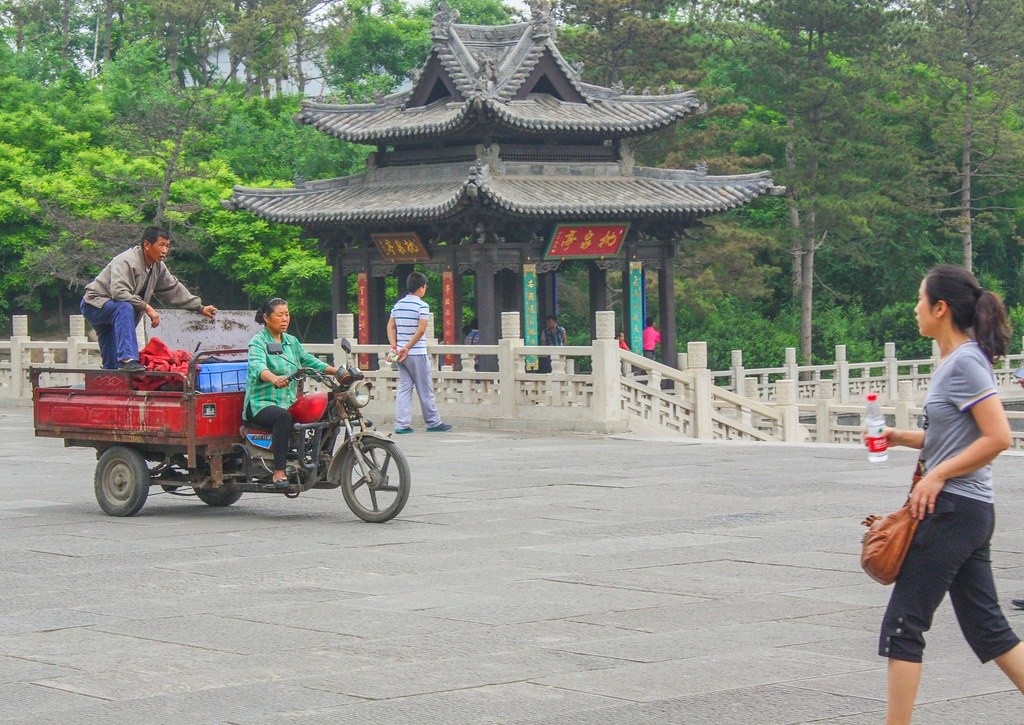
33, 338, 412, 524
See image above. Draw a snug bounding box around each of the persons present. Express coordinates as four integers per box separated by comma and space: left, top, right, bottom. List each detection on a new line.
642, 317, 661, 361
541, 315, 567, 347
242, 297, 340, 488
386, 271, 452, 434
863, 264, 1024, 725
438, 317, 481, 371
615, 331, 631, 352
80, 225, 219, 372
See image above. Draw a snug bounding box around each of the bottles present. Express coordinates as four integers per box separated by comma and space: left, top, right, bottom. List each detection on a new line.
861, 393, 889, 464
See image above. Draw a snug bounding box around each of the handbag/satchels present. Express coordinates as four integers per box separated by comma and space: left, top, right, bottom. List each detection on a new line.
862, 477, 921, 586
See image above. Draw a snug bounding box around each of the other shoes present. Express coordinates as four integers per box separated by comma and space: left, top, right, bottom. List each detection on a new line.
117, 359, 145, 371
427, 423, 452, 432
1011, 599, 1024, 607
395, 427, 414, 434
272, 475, 289, 488
